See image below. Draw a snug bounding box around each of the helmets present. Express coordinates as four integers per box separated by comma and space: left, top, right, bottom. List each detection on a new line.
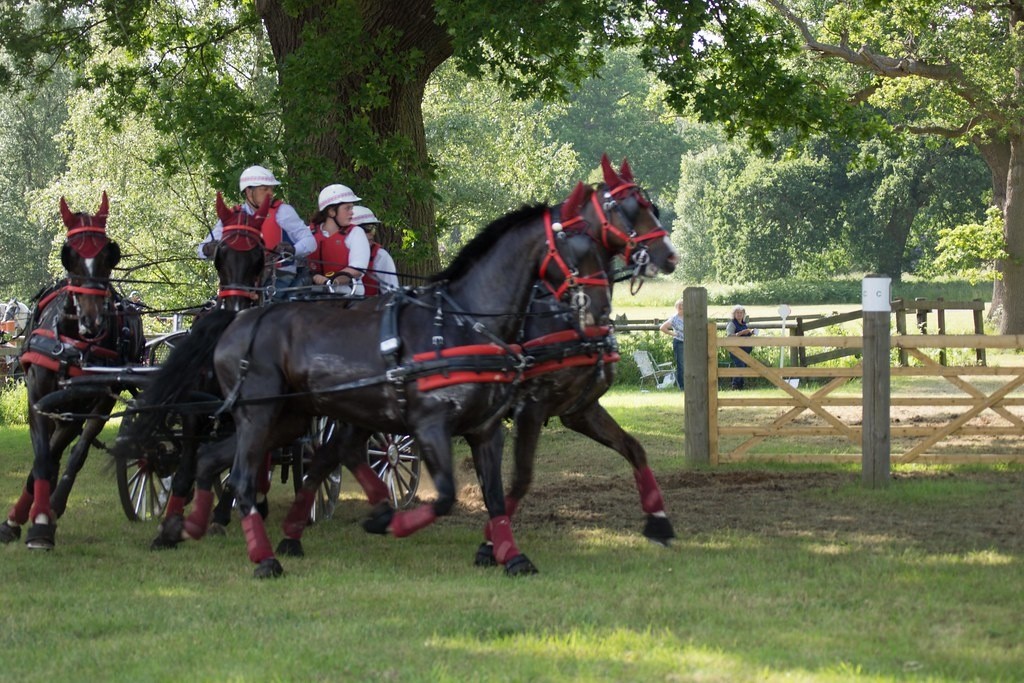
239, 165, 282, 192
318, 184, 363, 212
350, 205, 382, 225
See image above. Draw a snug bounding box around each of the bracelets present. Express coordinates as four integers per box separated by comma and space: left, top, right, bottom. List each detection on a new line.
736, 333, 739, 336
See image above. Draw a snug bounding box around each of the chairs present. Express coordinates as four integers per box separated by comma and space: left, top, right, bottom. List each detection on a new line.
631, 351, 681, 393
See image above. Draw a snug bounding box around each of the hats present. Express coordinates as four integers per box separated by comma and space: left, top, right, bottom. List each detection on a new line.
129, 291, 140, 298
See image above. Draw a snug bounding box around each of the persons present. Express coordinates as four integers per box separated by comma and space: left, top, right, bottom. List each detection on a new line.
127, 291, 143, 312
296, 184, 370, 311
349, 206, 399, 299
659, 298, 684, 391
726, 305, 754, 391
198, 165, 317, 303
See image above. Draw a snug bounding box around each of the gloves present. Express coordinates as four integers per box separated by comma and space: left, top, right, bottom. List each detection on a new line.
274, 240, 296, 256
203, 240, 218, 257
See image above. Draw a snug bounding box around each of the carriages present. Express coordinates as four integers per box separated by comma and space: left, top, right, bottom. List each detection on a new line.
0, 150, 682, 582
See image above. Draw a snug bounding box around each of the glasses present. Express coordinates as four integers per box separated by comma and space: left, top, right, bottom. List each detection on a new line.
362, 225, 375, 233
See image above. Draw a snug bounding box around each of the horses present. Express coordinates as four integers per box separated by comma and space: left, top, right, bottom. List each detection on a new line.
0, 188, 145, 554
137, 203, 604, 578
275, 156, 681, 549
